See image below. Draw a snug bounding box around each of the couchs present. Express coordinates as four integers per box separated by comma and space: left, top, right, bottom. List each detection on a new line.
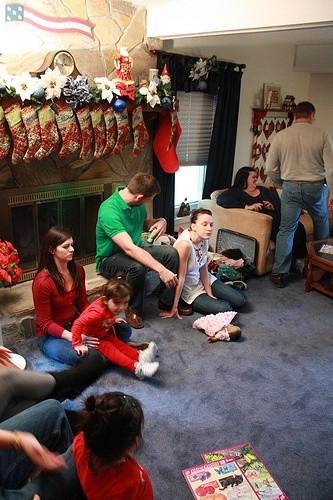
209, 189, 333, 277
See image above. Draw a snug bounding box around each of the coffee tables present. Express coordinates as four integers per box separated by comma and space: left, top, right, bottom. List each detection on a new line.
303, 241, 333, 298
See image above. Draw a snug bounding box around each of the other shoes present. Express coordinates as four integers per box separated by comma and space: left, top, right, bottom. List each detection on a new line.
295, 258, 306, 273
224, 280, 247, 290
269, 273, 285, 288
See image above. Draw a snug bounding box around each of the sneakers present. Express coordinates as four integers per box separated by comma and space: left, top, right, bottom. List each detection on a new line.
138, 341, 158, 364
135, 359, 160, 378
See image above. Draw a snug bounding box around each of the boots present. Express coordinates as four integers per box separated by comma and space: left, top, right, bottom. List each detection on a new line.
37, 350, 105, 403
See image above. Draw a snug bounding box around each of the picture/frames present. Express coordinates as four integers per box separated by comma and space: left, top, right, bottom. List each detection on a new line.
264, 84, 282, 108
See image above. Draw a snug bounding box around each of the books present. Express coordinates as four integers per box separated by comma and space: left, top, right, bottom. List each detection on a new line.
181, 456, 261, 500
201, 442, 290, 500
318, 245, 333, 255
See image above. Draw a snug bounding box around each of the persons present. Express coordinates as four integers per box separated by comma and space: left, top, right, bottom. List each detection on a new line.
72, 272, 160, 380
31, 226, 132, 366
216, 166, 307, 275
159, 209, 247, 319
192, 311, 241, 341
0, 344, 154, 500
96, 172, 192, 329
266, 101, 333, 287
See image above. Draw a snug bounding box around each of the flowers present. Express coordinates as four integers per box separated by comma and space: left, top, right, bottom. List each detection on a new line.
0, 62, 172, 109
189, 55, 214, 80
0, 240, 23, 289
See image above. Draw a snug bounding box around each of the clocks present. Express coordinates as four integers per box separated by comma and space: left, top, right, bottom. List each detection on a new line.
29, 49, 82, 80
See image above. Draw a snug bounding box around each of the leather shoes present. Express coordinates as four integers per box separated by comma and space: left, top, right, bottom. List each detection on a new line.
124, 305, 144, 329
158, 297, 192, 315
